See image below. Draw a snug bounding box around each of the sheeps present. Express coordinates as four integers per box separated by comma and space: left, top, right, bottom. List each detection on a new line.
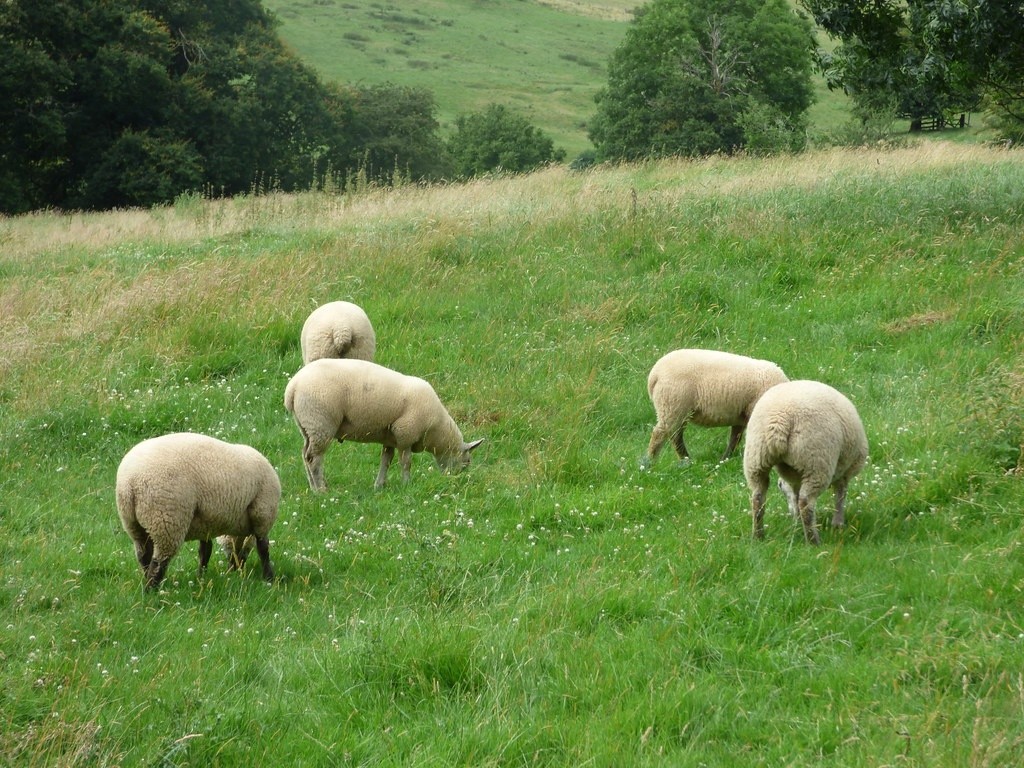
641, 348, 792, 469
743, 379, 869, 546
299, 299, 376, 368
285, 358, 484, 498
115, 432, 282, 597
